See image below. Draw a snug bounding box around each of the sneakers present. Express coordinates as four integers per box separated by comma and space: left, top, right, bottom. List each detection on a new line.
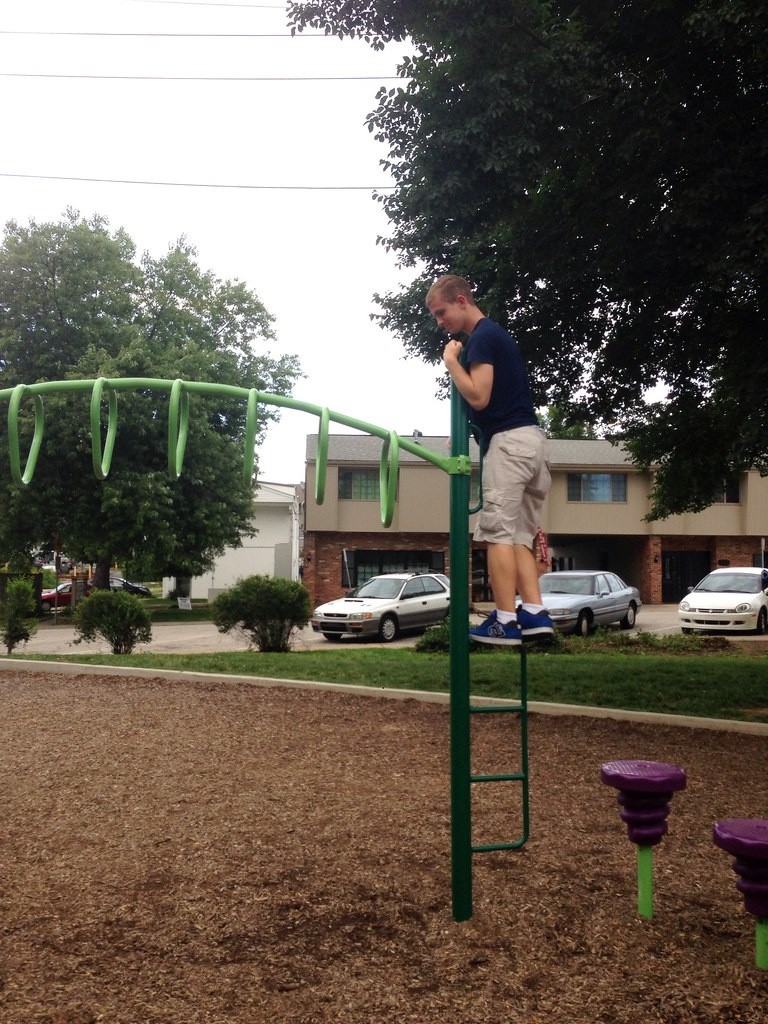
469, 611, 522, 645
515, 604, 554, 636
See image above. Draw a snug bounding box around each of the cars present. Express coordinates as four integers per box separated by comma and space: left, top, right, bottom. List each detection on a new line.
516, 570, 643, 636
471, 569, 492, 590
678, 567, 768, 637
40, 582, 95, 612
90, 577, 152, 598
308, 572, 449, 644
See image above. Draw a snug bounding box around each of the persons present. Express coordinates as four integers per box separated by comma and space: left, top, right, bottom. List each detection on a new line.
426, 274, 554, 644
